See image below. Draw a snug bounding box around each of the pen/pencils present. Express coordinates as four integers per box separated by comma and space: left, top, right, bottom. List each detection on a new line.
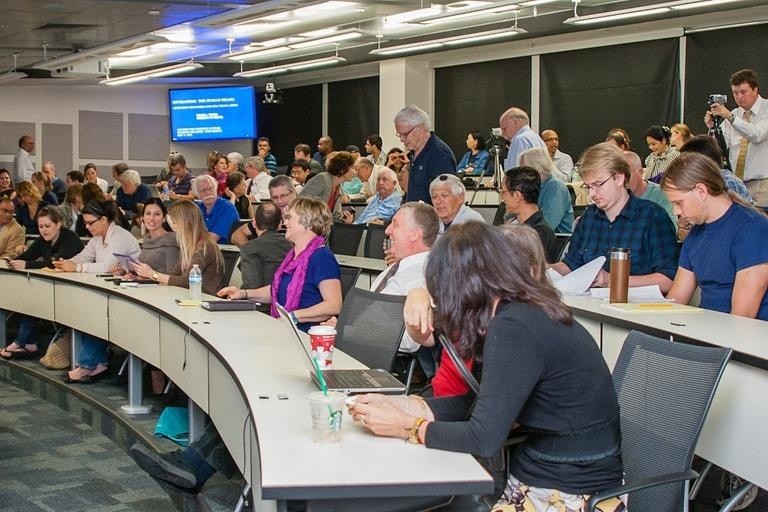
96, 274, 114, 277
104, 279, 121, 281
640, 303, 672, 307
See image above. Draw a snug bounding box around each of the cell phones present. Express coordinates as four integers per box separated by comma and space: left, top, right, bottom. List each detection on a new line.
3, 257, 12, 267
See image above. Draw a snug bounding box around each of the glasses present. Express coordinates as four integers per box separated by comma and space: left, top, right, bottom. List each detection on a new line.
82, 217, 102, 225
580, 175, 614, 192
500, 190, 512, 194
200, 187, 214, 191
544, 138, 559, 142
396, 127, 416, 138
428, 175, 463, 191
271, 192, 290, 200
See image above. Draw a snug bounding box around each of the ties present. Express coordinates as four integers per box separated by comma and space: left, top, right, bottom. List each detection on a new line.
374, 260, 399, 293
734, 111, 751, 182
246, 181, 253, 195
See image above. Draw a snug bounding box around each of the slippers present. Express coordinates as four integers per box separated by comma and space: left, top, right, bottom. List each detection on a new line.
0, 346, 22, 360
12, 346, 41, 358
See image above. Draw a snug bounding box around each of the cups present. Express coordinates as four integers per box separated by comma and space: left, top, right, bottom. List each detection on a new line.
306, 326, 338, 371
609, 247, 632, 304
309, 390, 346, 444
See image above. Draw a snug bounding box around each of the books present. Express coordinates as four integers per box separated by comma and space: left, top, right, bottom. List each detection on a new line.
174, 298, 256, 311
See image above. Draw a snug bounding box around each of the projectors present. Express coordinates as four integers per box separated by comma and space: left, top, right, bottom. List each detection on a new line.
50, 55, 109, 82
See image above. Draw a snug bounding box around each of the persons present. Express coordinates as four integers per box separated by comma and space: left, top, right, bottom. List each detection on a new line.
0, 124, 768, 403
704, 68, 768, 215
130, 195, 341, 498
497, 107, 549, 179
383, 104, 457, 267
347, 218, 628, 512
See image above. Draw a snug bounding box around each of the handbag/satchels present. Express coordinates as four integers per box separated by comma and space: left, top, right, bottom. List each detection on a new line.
154, 407, 190, 446
40, 335, 72, 369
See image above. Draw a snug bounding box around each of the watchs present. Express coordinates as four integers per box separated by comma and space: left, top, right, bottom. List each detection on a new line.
408, 416, 426, 445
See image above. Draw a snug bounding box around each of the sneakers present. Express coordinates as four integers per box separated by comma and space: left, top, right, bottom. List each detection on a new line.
131, 442, 201, 498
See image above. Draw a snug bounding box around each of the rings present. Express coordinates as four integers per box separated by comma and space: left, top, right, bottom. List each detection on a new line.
360, 416, 366, 425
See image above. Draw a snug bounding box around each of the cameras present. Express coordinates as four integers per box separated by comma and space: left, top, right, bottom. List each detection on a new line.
708, 94, 729, 106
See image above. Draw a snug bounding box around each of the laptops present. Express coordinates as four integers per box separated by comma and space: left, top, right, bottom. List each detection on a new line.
147, 184, 168, 200
274, 303, 408, 395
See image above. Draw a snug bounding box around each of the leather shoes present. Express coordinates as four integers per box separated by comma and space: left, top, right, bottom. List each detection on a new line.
58, 373, 78, 383
78, 369, 112, 383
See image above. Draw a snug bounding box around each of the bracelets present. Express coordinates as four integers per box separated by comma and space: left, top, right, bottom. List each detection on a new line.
416, 398, 426, 417
401, 397, 409, 415
727, 110, 734, 120
243, 290, 248, 300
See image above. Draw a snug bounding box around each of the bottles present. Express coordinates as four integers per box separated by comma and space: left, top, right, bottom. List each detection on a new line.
188, 264, 203, 302
162, 184, 169, 202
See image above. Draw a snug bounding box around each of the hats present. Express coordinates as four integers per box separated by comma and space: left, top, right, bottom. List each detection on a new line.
347, 146, 359, 153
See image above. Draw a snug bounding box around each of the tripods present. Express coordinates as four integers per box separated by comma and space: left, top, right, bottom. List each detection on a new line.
467, 136, 507, 209
